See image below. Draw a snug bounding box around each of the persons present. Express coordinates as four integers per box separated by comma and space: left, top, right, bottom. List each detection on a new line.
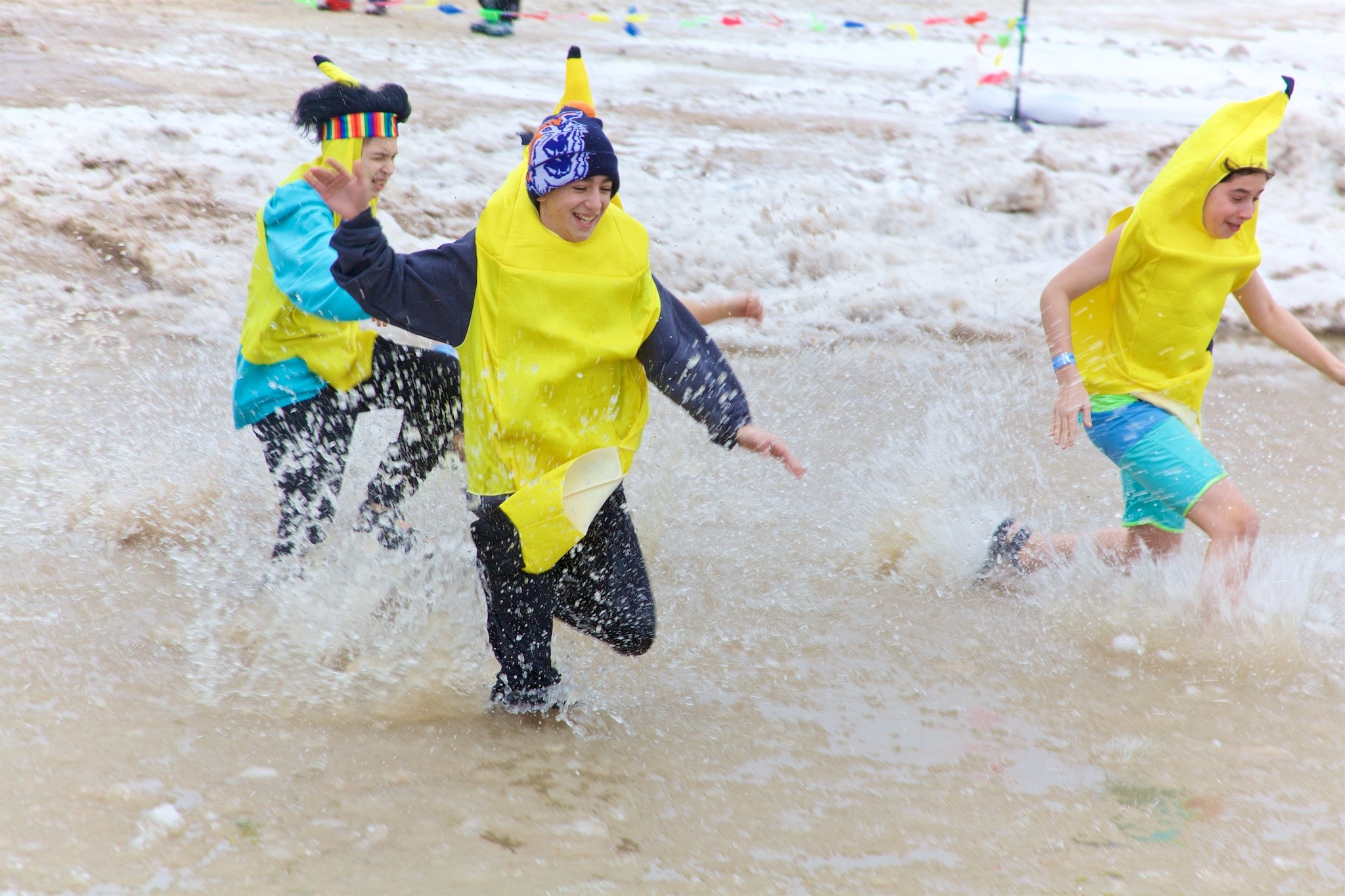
227, 52, 463, 573
303, 40, 810, 721
967, 76, 1345, 613
318, 0, 393, 14
469, 0, 521, 38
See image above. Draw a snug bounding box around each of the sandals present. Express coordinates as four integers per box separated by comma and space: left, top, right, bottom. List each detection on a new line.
970, 518, 1031, 587
354, 500, 429, 551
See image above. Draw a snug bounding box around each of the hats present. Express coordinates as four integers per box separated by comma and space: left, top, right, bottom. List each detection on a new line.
526, 107, 620, 211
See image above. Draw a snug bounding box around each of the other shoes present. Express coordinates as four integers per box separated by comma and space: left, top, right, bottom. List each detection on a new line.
366, 4, 386, 13
319, 2, 332, 10
471, 19, 496, 32
486, 21, 512, 34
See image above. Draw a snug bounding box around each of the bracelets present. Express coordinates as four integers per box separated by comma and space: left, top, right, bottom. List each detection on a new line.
1051, 352, 1076, 370
